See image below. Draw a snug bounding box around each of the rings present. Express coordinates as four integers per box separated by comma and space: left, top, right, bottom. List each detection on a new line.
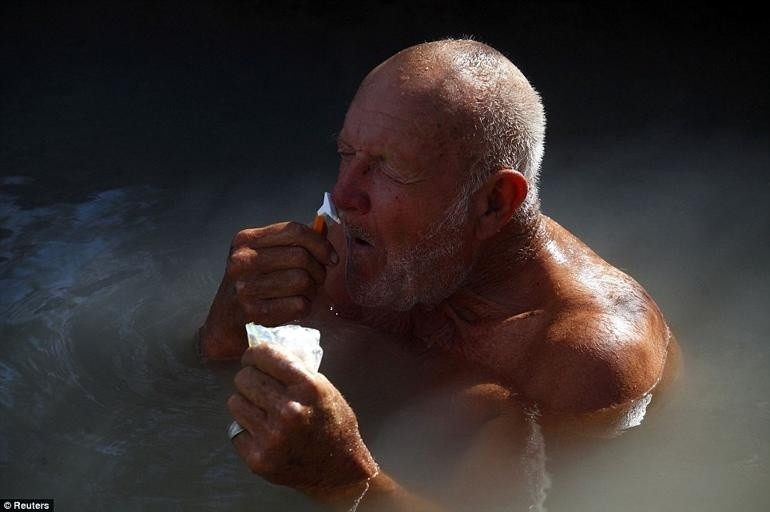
227, 422, 245, 443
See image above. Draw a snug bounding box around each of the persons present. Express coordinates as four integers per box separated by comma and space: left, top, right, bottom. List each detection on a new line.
199, 40, 681, 510
